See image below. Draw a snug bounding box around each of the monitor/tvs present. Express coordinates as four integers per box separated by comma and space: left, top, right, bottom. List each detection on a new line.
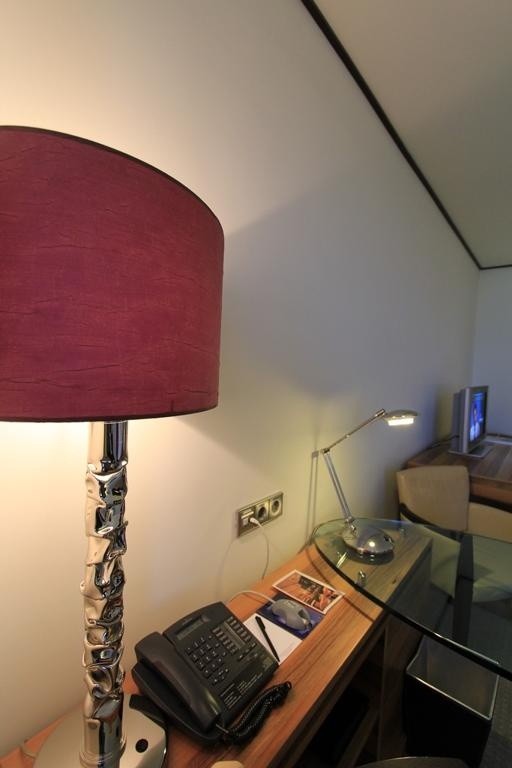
448, 384, 492, 458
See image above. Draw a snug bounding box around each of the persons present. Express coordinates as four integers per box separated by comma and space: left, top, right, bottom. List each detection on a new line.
469, 403, 481, 442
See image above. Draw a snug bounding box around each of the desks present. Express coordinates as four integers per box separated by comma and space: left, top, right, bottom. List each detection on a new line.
4, 518, 433, 764
312, 515, 511, 684
402, 432, 510, 509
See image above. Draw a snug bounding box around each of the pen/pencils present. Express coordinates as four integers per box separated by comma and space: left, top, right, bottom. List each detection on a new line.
255, 616, 280, 662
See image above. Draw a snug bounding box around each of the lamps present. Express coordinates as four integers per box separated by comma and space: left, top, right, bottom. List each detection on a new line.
2, 125, 223, 764
319, 408, 418, 559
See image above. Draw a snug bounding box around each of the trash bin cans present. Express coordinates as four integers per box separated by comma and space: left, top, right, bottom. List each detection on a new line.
405, 632, 500, 768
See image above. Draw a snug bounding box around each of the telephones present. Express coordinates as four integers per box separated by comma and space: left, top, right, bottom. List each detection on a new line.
131, 602, 280, 750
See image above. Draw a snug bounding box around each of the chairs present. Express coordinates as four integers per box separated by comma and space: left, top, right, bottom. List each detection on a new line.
396, 463, 512, 603
